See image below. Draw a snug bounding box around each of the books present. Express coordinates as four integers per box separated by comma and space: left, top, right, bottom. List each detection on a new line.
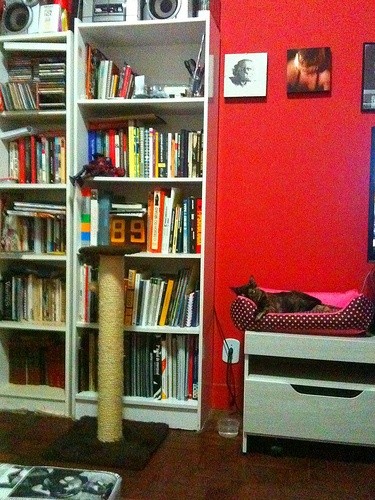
76, 331, 199, 405
7, 332, 65, 391
0, 119, 66, 185
0, 56, 66, 113
0, 191, 67, 255
80, 259, 200, 328
0, 267, 66, 324
84, 45, 136, 98
87, 112, 203, 178
79, 186, 201, 255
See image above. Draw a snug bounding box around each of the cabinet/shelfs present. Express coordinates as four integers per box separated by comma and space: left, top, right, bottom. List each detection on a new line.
0, 28, 74, 416
71, 8, 222, 434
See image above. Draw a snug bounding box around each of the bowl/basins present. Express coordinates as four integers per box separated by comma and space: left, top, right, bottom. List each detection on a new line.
217, 418, 240, 438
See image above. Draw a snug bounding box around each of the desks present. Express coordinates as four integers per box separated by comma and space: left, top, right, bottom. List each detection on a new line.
241, 328, 375, 454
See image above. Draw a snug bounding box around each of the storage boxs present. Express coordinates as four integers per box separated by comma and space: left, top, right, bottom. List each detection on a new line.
39, 4, 64, 33
27, 3, 41, 34
134, 73, 146, 95
164, 86, 192, 98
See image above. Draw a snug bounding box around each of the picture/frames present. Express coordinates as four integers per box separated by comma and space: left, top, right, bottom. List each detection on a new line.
359, 41, 375, 113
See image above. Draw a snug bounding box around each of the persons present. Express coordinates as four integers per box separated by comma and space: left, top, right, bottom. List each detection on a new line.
287, 48, 331, 91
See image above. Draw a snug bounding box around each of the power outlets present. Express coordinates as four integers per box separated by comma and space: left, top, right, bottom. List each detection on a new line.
221, 338, 240, 364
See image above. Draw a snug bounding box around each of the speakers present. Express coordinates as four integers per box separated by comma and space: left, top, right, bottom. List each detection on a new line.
142, 0, 203, 20
1, 0, 49, 33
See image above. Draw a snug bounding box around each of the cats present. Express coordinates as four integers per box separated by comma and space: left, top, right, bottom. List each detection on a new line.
229, 274, 342, 321
